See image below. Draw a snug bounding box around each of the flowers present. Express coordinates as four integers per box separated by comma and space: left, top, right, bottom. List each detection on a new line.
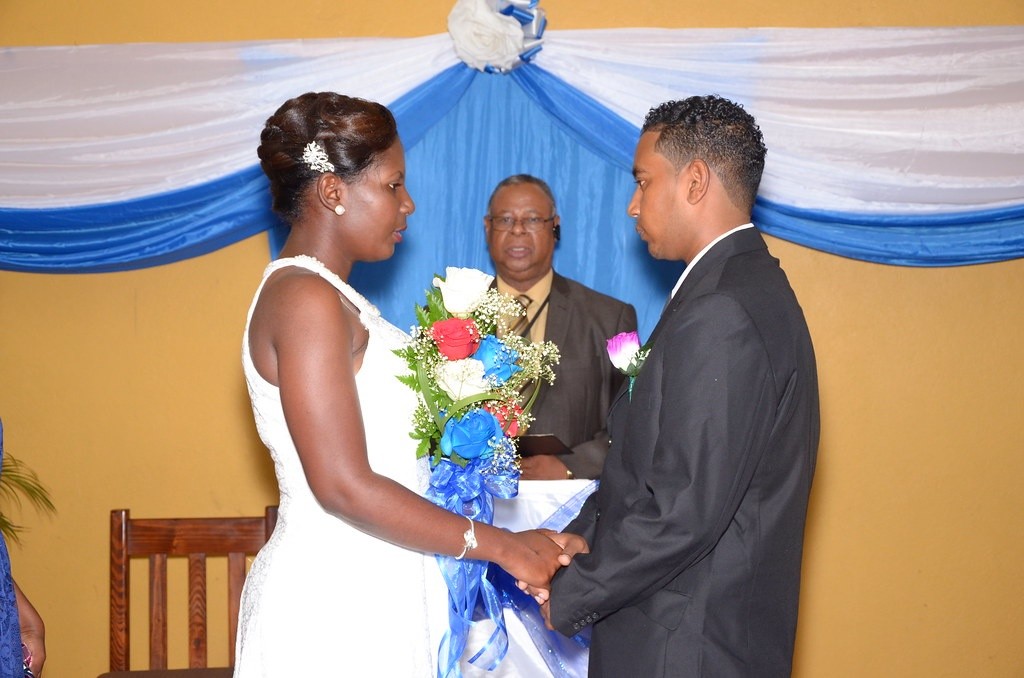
605, 332, 651, 403
391, 266, 588, 678
443, 1, 549, 73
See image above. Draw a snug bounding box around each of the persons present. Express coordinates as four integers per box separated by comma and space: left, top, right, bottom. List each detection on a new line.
422, 174, 639, 480
515, 94, 822, 678
234, 89, 565, 678
0, 418, 45, 678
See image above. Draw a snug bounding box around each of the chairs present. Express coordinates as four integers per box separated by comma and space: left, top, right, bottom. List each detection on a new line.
90, 505, 282, 678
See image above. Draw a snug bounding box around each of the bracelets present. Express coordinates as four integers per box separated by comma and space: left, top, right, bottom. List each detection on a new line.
455, 514, 478, 560
567, 468, 574, 479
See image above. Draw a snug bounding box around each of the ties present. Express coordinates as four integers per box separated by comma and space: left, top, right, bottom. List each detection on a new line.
507, 294, 535, 410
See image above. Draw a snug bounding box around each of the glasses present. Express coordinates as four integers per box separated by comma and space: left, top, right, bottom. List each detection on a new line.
488, 214, 555, 232
